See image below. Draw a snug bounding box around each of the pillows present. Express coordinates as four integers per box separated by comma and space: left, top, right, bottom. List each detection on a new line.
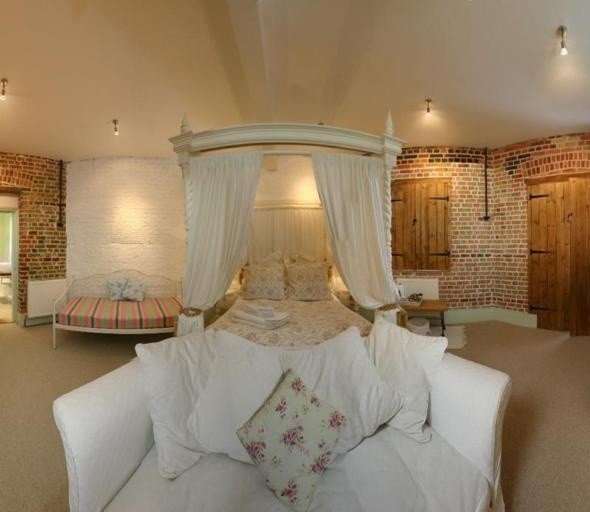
284, 261, 332, 301
363, 317, 448, 443
236, 368, 348, 512
279, 325, 402, 454
51, 337, 513, 512
241, 264, 285, 301
107, 277, 127, 301
122, 278, 145, 302
175, 330, 283, 465
136, 329, 217, 479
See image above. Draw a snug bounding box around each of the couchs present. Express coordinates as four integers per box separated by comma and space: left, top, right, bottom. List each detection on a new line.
51, 269, 183, 348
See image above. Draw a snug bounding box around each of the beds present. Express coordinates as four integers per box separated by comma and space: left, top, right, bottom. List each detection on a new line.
167, 109, 409, 349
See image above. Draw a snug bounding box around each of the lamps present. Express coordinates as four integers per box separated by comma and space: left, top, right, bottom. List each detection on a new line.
113, 120, 120, 136
424, 98, 432, 115
0, 79, 9, 100
557, 25, 569, 56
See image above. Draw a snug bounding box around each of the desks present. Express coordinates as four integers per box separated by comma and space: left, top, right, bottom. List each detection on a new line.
399, 299, 449, 336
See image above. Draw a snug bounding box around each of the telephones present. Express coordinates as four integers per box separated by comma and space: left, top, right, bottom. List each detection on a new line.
398, 283, 407, 301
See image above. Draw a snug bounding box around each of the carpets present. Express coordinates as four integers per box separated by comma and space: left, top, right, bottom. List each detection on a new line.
427, 324, 467, 349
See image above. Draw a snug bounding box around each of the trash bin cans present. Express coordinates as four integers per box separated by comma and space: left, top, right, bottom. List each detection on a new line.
406, 317, 430, 335
